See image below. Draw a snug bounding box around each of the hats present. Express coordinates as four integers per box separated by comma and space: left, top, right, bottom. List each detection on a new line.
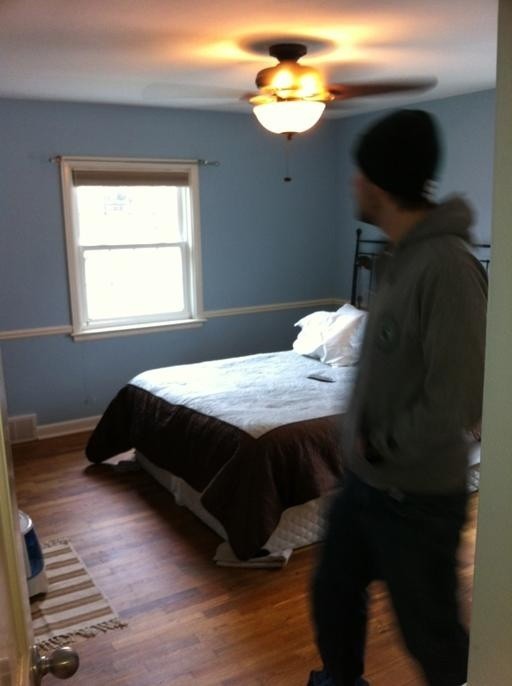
355, 109, 439, 196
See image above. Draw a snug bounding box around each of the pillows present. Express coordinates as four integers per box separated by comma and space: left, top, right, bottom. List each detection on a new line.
292, 301, 369, 366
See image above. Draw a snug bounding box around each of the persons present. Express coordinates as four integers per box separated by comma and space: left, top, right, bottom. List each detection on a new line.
307, 107, 491, 686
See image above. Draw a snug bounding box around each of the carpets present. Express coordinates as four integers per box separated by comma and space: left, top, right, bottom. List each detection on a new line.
27, 533, 127, 653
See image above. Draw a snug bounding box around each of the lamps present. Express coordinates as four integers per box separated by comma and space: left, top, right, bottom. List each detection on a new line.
249, 66, 329, 140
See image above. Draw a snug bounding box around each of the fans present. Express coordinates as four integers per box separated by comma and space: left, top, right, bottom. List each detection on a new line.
137, 43, 437, 144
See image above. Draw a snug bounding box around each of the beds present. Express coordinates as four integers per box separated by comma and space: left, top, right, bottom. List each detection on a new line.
84, 227, 495, 569
